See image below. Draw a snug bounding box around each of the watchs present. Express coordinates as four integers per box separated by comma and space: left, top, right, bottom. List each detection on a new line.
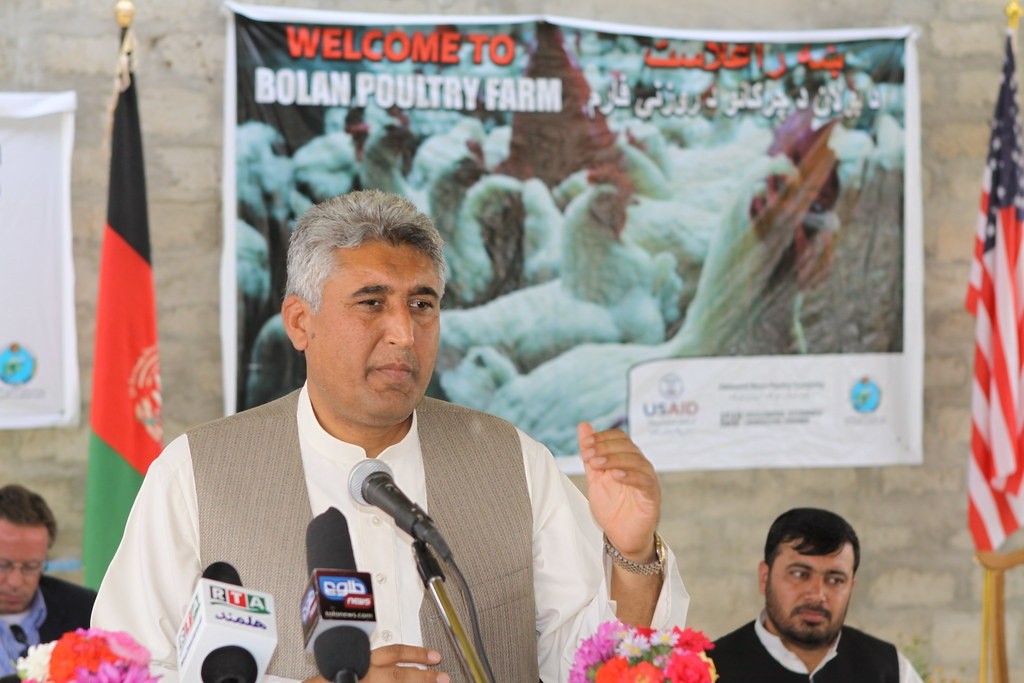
603, 531, 666, 576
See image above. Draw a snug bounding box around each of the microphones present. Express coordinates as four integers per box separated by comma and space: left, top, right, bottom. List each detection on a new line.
175, 561, 278, 683
347, 458, 452, 564
9, 624, 30, 647
299, 508, 378, 683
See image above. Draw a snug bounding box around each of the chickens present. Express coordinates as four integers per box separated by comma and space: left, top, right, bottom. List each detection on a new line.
233, 19, 907, 454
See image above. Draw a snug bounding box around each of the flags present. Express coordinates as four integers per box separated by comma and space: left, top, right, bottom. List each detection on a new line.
966, 29, 1022, 556
86, 29, 169, 596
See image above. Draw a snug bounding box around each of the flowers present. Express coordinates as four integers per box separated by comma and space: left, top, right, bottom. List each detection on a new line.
8, 627, 167, 683
567, 618, 719, 683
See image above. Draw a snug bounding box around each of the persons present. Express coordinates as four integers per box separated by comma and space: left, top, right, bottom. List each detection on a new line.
91, 187, 688, 682
696, 508, 929, 683
0, 484, 99, 683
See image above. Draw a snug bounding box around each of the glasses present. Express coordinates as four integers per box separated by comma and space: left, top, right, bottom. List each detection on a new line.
10, 624, 33, 659
